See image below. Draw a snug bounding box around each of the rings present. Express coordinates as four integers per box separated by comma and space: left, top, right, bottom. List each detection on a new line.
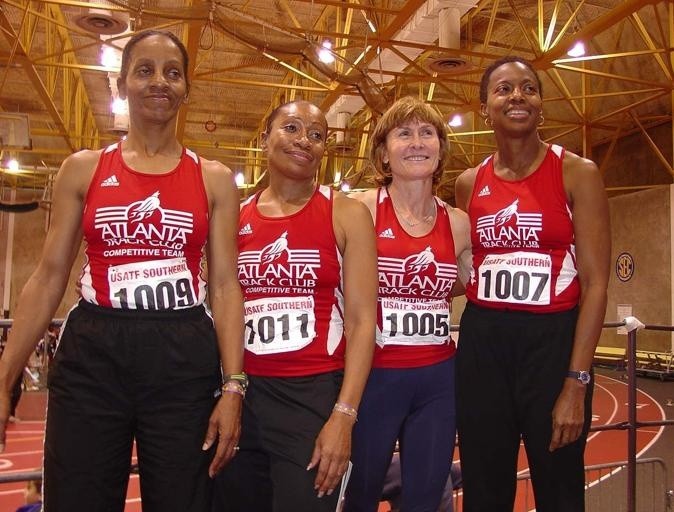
233, 445, 239, 450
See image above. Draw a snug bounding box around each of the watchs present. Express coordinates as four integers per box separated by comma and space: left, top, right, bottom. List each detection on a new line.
564, 369, 591, 385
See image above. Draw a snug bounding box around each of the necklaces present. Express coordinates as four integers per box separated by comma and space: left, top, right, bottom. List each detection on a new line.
391, 203, 435, 228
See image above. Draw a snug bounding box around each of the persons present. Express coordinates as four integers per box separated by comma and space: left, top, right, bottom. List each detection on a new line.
15, 478, 41, 512
454, 54, 612, 512
0, 30, 253, 512
335, 95, 478, 512
200, 100, 378, 512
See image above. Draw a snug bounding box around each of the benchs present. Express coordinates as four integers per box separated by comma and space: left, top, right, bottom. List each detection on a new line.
627, 352, 673, 381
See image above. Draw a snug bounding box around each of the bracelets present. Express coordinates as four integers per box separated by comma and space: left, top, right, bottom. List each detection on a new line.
331, 400, 359, 424
218, 382, 246, 399
221, 371, 249, 388
